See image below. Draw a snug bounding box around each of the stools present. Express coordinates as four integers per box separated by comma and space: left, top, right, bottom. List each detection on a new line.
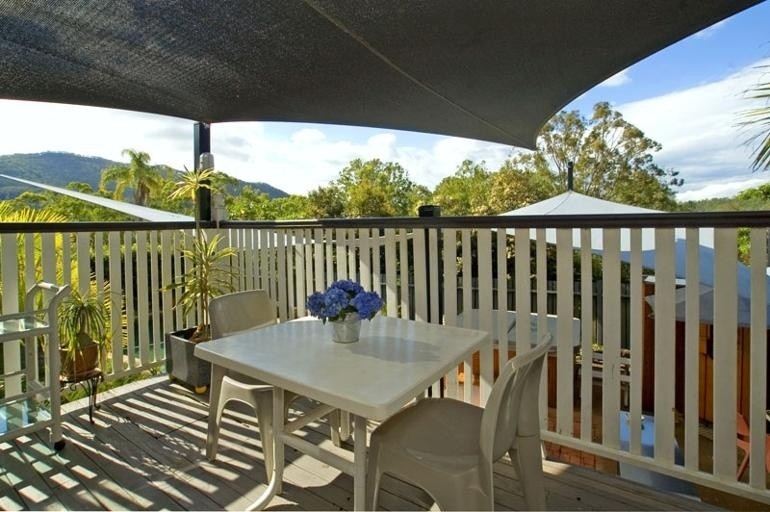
59, 368, 105, 426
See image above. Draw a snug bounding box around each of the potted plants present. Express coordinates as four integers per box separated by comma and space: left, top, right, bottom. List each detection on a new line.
56, 286, 121, 378
153, 229, 241, 394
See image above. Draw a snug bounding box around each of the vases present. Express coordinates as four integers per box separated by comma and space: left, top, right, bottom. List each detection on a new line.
331, 312, 361, 343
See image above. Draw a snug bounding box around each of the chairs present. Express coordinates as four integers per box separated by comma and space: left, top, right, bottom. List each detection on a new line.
365, 330, 553, 511
207, 289, 342, 494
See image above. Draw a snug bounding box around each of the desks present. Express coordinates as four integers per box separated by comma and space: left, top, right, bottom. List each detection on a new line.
193, 303, 493, 512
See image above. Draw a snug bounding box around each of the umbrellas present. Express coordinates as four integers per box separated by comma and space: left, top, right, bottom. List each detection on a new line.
646, 275, 770, 332
487, 190, 761, 299
1, 0, 770, 152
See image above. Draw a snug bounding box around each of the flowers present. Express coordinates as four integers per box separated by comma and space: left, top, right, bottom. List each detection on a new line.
305, 279, 383, 325
